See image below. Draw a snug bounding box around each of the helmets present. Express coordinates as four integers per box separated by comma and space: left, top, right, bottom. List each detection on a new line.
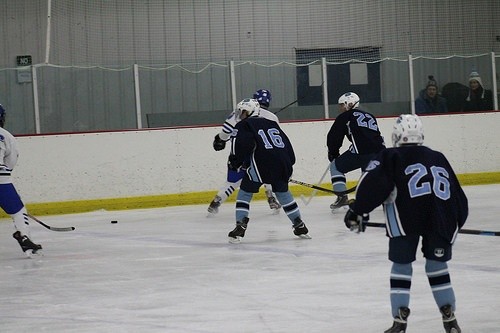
0, 103, 6, 127
236, 98, 260, 117
338, 92, 360, 111
391, 114, 424, 148
253, 89, 272, 107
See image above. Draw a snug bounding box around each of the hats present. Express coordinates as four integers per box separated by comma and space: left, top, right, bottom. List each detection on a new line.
426, 76, 437, 90
465, 72, 485, 101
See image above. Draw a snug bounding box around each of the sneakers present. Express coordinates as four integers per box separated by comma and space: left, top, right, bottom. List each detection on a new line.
206, 194, 222, 219
384, 306, 410, 333
228, 217, 249, 244
329, 194, 350, 214
13, 230, 44, 258
291, 219, 311, 239
268, 196, 281, 214
439, 305, 461, 333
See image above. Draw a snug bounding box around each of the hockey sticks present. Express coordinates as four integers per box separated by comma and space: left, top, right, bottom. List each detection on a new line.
28, 213, 75, 232
273, 81, 324, 114
289, 178, 358, 196
301, 161, 332, 206
365, 221, 500, 236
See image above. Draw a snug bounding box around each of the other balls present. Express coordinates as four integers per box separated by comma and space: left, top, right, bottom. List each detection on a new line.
111, 220, 117, 223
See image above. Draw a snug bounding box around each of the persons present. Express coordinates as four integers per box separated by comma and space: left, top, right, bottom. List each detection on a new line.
207, 88, 281, 215
344, 113, 468, 333
227, 98, 312, 243
461, 71, 494, 111
0, 103, 42, 258
326, 92, 387, 214
415, 75, 448, 115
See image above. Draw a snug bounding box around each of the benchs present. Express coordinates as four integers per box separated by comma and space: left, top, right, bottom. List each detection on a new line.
145, 100, 412, 129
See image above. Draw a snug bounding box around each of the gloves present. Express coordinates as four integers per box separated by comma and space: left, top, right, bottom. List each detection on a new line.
328, 149, 340, 163
344, 199, 369, 233
213, 134, 226, 151
228, 154, 242, 171
